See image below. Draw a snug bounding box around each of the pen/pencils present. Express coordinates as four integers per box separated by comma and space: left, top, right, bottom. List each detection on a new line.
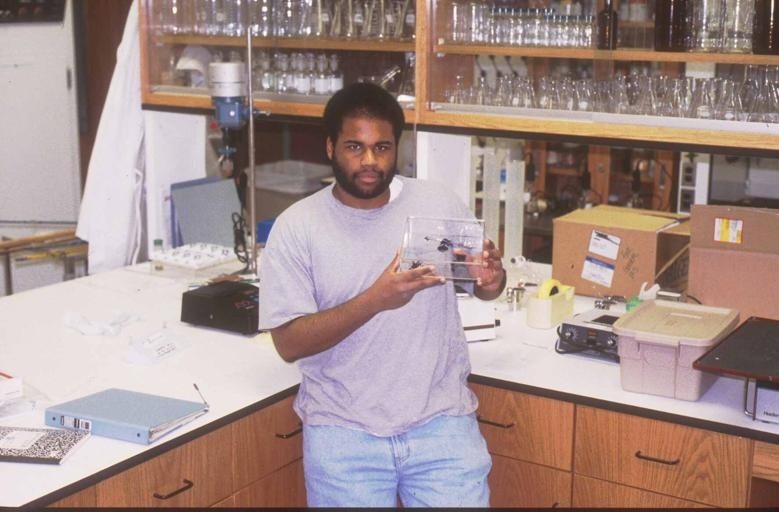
194, 384, 207, 405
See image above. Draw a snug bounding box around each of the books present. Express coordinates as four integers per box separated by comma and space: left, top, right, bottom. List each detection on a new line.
0, 426, 91, 464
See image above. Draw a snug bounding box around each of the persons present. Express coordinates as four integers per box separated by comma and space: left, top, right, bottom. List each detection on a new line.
258, 83, 506, 508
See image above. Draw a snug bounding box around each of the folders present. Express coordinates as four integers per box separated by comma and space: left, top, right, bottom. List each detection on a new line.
44, 388, 207, 444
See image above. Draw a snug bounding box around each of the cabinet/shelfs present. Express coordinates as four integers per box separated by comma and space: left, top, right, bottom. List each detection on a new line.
421, 0, 779, 158
460, 382, 779, 509
137, 0, 416, 131
46, 394, 313, 509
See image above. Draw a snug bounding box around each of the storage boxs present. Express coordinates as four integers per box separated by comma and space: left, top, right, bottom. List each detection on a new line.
552, 205, 690, 301
611, 298, 739, 404
689, 202, 779, 324
244, 159, 334, 218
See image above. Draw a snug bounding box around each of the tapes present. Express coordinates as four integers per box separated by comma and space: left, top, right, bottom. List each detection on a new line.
538, 278, 562, 299
